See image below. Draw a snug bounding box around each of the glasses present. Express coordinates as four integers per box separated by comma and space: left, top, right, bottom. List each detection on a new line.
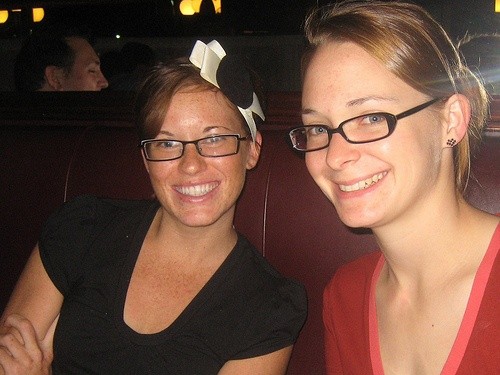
137, 133, 247, 162
286, 95, 443, 153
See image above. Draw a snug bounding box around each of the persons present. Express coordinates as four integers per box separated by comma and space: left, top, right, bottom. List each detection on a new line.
12, 27, 109, 92
287, 0, 500, 375
0, 55, 309, 375
